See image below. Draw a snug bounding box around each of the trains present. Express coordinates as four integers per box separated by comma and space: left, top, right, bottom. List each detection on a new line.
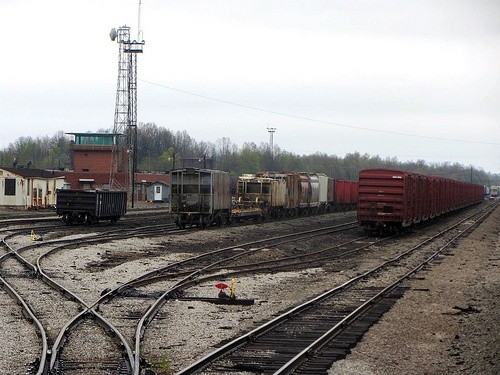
358, 169, 485, 237
56, 188, 129, 226
169, 168, 357, 229
